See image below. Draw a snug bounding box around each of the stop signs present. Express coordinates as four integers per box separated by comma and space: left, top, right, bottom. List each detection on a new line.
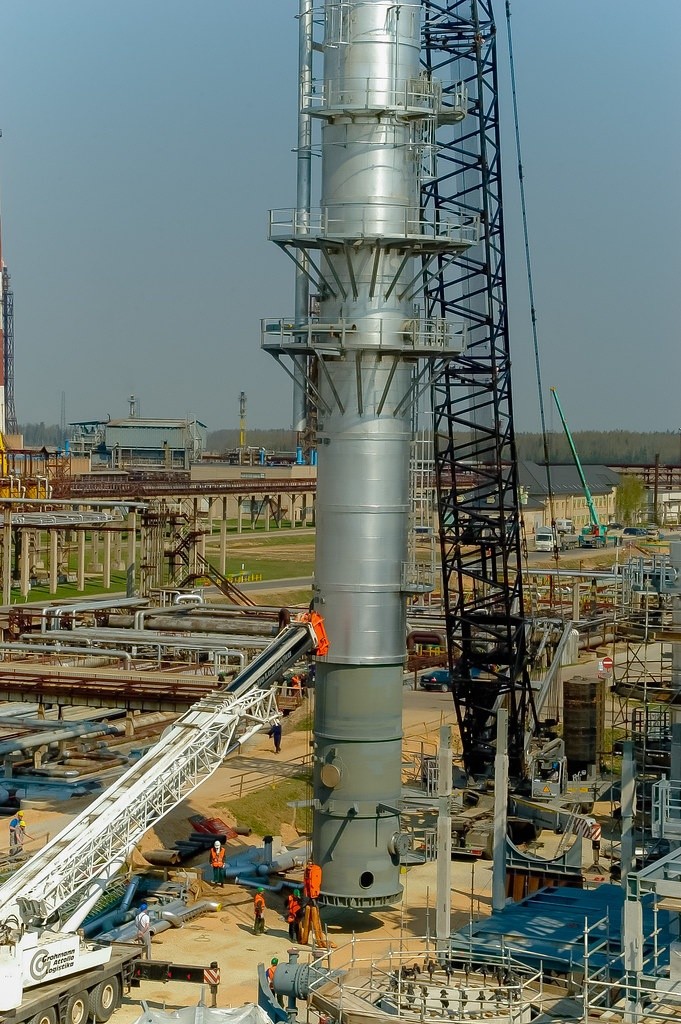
602, 658, 612, 668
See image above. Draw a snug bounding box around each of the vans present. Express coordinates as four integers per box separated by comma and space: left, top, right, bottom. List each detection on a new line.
557, 520, 574, 534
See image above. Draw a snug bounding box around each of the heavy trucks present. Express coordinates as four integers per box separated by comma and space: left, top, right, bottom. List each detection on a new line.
535, 531, 578, 551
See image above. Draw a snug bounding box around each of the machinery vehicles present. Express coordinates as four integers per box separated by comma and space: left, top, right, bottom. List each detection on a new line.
0, 611, 329, 1024
548, 386, 608, 549
421, 0, 569, 865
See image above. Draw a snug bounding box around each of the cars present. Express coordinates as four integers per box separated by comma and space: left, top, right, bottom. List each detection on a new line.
419, 670, 453, 693
623, 526, 647, 537
606, 523, 623, 530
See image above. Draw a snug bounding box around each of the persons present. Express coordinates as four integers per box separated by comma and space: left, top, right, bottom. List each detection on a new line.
209, 841, 227, 889
266, 957, 285, 1010
284, 888, 305, 943
253, 886, 267, 935
9, 811, 24, 846
269, 719, 282, 754
135, 904, 151, 960
16, 821, 35, 845
